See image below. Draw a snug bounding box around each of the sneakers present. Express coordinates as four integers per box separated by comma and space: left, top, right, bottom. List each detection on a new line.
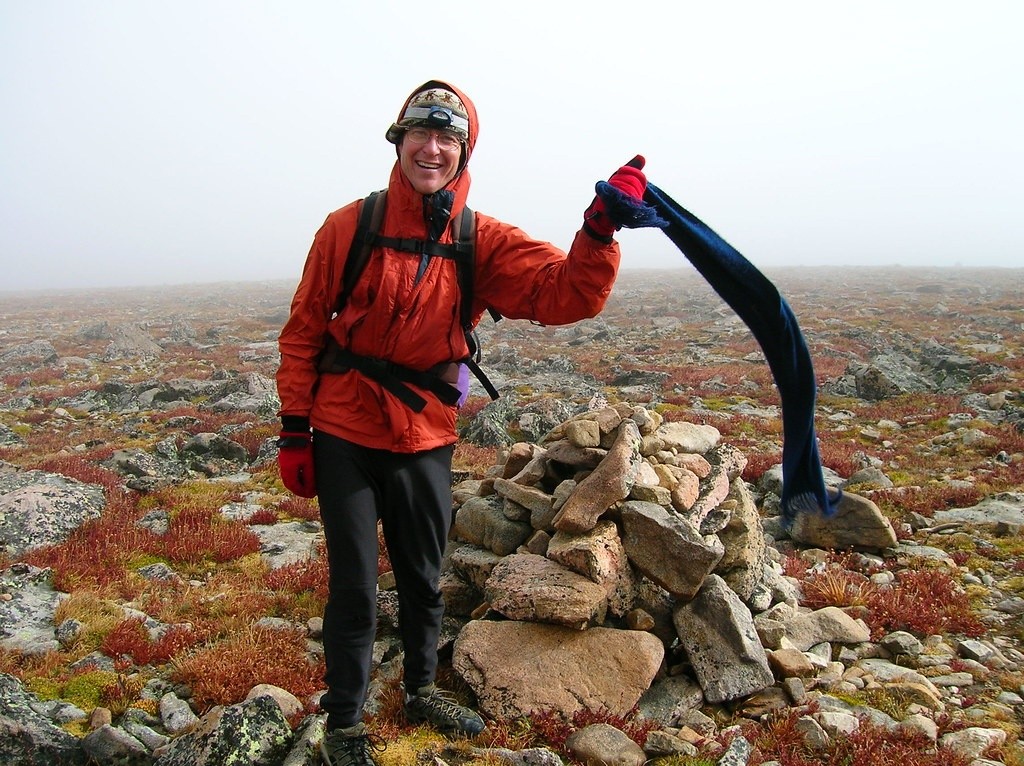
402, 681, 485, 737
319, 722, 376, 766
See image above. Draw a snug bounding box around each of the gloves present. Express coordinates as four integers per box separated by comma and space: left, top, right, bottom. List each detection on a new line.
584, 155, 647, 238
276, 431, 318, 499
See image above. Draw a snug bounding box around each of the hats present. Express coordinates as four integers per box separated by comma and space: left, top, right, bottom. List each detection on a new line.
385, 87, 469, 180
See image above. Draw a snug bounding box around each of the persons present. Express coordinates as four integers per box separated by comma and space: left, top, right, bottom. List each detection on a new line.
277, 79, 647, 766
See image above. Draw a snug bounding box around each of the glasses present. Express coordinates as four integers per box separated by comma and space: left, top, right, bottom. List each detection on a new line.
405, 126, 464, 152
